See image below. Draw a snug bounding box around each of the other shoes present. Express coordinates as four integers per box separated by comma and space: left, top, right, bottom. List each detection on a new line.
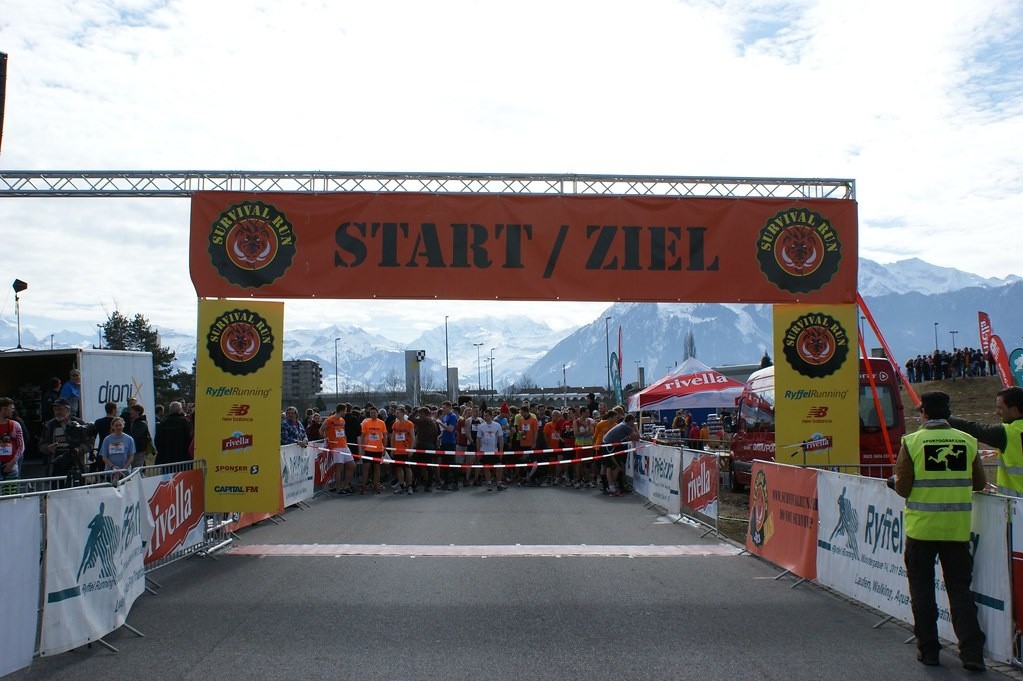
915, 648, 939, 664
963, 657, 986, 672
336, 480, 626, 497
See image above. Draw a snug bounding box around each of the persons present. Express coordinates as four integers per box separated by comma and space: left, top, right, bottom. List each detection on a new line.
887, 388, 987, 672
916, 388, 1023, 498
0, 370, 747, 496
895, 347, 997, 383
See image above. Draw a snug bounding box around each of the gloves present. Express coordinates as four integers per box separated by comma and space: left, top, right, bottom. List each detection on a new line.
887, 475, 895, 490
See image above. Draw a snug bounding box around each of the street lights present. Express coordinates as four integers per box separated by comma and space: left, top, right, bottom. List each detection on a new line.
484, 347, 495, 400
934, 322, 939, 350
334, 337, 340, 403
860, 316, 866, 341
607, 316, 612, 399
949, 330, 959, 351
12, 279, 28, 348
445, 316, 449, 395
473, 343, 483, 396
96, 323, 105, 349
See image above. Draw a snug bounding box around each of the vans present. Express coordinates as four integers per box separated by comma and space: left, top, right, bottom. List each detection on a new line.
722, 357, 906, 494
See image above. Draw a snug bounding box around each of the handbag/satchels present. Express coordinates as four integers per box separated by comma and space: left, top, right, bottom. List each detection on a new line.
146, 437, 155, 454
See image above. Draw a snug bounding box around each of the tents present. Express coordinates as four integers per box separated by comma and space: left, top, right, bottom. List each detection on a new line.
628, 356, 745, 434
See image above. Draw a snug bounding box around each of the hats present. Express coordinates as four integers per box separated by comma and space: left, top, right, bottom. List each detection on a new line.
49, 377, 61, 389
587, 393, 595, 400
917, 392, 950, 411
676, 416, 684, 427
51, 398, 70, 407
500, 403, 510, 413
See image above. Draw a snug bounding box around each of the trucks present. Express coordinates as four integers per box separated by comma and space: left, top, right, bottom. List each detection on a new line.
0, 348, 156, 473
